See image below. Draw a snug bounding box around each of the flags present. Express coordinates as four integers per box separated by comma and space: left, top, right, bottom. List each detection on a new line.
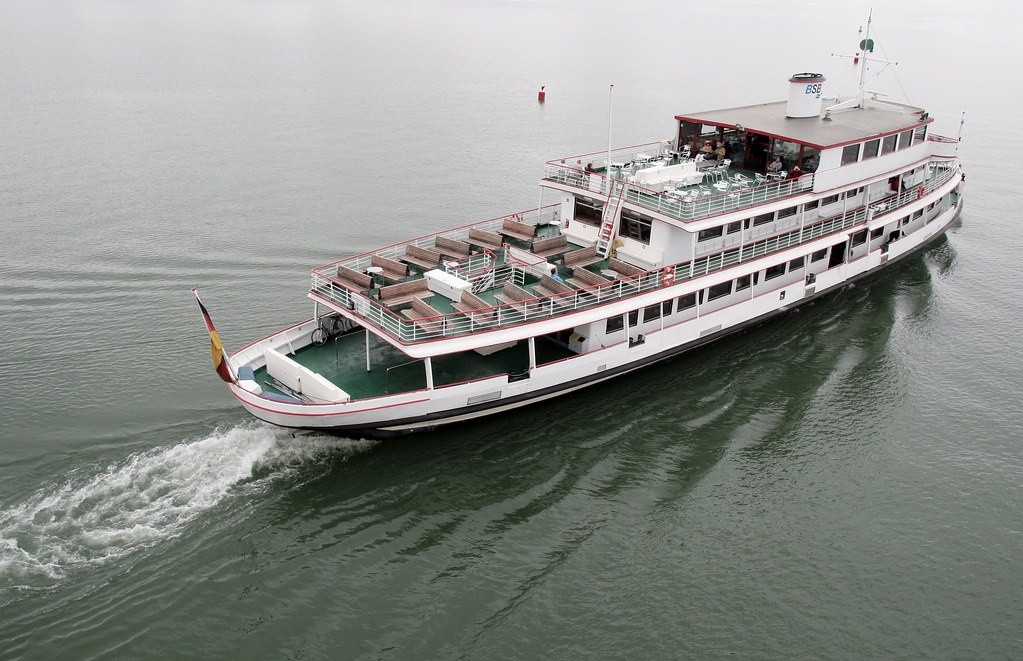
197, 298, 235, 383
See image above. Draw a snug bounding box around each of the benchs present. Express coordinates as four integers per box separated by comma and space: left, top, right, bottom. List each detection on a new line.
399, 296, 458, 336
565, 266, 612, 299
600, 256, 650, 287
398, 244, 440, 270
629, 161, 706, 195
450, 289, 505, 326
422, 269, 472, 302
530, 235, 575, 262
560, 220, 664, 266
530, 274, 586, 307
463, 227, 512, 253
428, 236, 478, 258
493, 280, 549, 316
334, 255, 418, 298
553, 245, 605, 275
496, 219, 536, 247
372, 277, 435, 308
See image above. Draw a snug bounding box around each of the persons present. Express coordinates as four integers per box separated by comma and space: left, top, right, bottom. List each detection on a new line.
789, 166, 803, 180
698, 141, 727, 160
550, 268, 564, 282
770, 157, 783, 171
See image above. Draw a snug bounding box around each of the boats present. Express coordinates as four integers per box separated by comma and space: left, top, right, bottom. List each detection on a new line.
193, 10, 970, 442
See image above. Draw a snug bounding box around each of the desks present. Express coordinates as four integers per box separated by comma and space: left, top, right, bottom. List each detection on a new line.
612, 162, 629, 180
547, 220, 561, 238
695, 160, 718, 183
669, 151, 681, 164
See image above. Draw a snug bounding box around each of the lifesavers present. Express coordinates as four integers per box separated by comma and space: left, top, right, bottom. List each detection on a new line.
660, 267, 674, 287
917, 187, 925, 199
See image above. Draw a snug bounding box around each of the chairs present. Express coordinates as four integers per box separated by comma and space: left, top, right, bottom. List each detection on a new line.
663, 171, 788, 210
603, 144, 692, 182
680, 157, 732, 183
557, 164, 568, 183
